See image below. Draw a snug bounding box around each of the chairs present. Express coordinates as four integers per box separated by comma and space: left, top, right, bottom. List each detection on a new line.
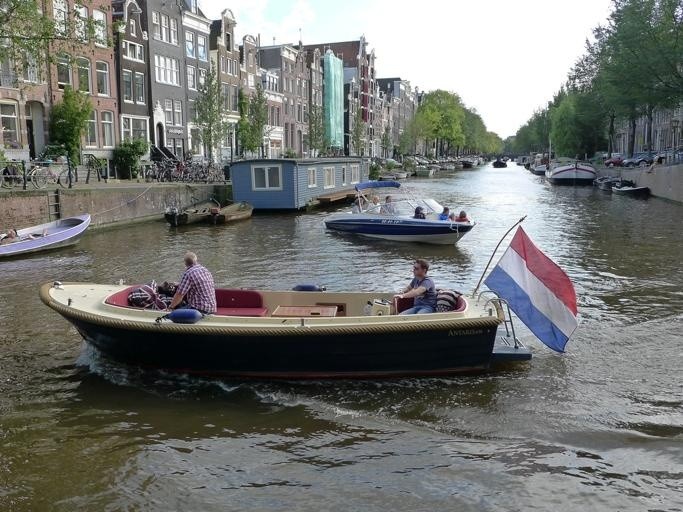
395, 297, 414, 315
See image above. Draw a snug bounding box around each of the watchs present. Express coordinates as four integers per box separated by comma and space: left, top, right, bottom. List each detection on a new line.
169, 305, 172, 309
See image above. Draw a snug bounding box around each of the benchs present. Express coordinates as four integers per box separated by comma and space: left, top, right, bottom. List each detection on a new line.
213, 308, 268, 317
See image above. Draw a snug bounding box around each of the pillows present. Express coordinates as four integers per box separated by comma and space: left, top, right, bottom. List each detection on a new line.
435, 291, 463, 312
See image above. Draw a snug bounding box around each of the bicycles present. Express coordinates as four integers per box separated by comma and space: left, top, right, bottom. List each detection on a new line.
0, 159, 76, 188
146, 158, 216, 183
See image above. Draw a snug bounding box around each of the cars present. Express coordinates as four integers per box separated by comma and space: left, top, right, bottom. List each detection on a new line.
602, 153, 657, 168
385, 156, 458, 168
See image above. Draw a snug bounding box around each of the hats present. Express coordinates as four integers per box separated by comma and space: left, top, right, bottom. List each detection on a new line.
415, 207, 424, 214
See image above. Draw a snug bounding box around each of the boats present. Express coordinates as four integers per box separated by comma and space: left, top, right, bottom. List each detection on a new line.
457, 155, 485, 166
493, 161, 507, 168
594, 176, 649, 197
164, 198, 253, 226
517, 154, 596, 184
41, 279, 532, 380
0, 213, 91, 257
324, 181, 475, 245
380, 163, 463, 180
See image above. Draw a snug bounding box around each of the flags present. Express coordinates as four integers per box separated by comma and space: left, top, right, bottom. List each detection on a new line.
482, 225, 577, 355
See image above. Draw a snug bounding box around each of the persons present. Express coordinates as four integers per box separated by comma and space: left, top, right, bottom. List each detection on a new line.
167, 252, 217, 315
380, 196, 394, 214
647, 154, 662, 173
367, 196, 381, 214
352, 198, 364, 213
456, 211, 468, 222
439, 207, 449, 220
393, 259, 436, 314
414, 206, 425, 218
4, 164, 22, 184
